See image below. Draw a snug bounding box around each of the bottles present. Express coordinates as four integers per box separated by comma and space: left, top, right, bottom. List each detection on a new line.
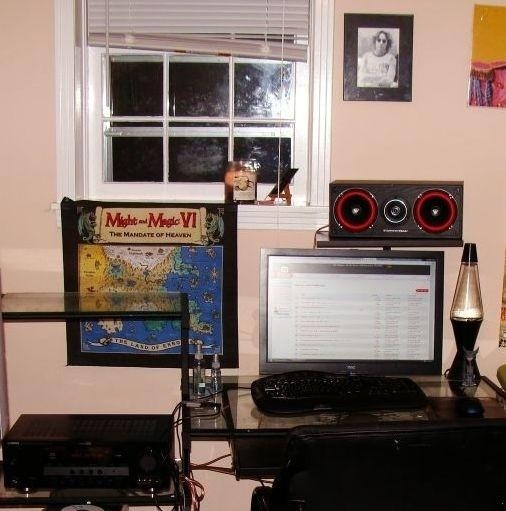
191, 339, 223, 393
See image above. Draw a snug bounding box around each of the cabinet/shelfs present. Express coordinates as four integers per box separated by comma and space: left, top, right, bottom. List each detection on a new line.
0, 292, 191, 511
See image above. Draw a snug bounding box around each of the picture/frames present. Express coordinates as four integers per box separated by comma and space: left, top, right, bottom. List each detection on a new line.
343, 12, 414, 103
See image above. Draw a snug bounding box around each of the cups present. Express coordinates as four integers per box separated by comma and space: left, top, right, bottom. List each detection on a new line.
225, 159, 260, 201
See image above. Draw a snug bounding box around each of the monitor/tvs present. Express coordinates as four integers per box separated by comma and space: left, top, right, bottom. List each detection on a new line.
259, 247, 444, 376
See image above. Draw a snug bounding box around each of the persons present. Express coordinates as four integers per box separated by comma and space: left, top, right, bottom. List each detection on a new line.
358, 30, 396, 87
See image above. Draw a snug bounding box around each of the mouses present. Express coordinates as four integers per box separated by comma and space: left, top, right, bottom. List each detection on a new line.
454, 397, 484, 418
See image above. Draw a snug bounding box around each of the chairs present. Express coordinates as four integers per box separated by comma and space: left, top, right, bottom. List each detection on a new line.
254, 420, 506, 511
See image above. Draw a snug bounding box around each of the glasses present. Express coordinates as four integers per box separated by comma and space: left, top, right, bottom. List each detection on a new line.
376, 39, 387, 43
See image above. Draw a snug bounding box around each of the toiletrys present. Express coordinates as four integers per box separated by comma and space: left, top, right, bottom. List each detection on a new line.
189, 338, 205, 397
209, 354, 222, 393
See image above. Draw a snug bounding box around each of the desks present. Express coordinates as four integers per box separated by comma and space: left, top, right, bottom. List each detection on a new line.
181, 374, 506, 481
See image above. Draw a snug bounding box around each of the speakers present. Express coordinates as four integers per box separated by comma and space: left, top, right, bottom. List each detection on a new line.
328, 180, 464, 241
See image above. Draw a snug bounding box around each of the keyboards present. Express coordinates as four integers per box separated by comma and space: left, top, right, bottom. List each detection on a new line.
251, 370, 428, 415
